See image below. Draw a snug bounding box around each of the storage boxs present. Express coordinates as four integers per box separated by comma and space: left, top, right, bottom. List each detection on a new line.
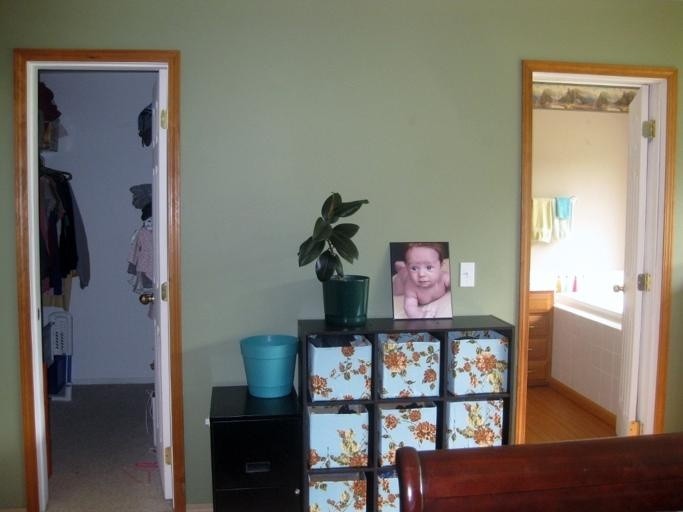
308, 331, 510, 512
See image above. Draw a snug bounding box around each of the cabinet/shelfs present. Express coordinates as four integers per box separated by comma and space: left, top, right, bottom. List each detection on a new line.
297, 314, 517, 511
526, 292, 554, 386
209, 385, 301, 511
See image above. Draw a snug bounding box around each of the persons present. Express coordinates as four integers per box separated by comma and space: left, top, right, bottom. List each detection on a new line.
389, 242, 451, 319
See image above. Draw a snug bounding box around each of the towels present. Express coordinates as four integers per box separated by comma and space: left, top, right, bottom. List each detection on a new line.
532, 197, 572, 246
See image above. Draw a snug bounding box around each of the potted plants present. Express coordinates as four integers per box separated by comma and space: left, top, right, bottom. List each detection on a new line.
295, 192, 371, 328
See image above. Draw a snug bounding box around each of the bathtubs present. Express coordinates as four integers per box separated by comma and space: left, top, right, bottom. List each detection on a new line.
551, 290, 620, 402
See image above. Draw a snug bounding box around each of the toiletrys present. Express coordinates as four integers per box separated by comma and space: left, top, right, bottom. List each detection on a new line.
563, 275, 570, 292
571, 276, 578, 293
556, 274, 561, 291
